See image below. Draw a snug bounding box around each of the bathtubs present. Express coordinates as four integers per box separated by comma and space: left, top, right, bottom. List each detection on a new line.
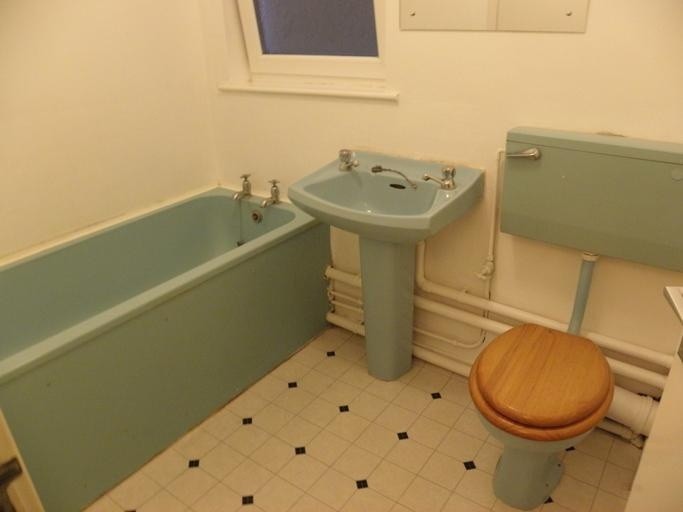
0, 186, 332, 512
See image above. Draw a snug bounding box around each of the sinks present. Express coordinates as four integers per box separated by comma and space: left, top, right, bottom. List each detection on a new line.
289, 150, 485, 243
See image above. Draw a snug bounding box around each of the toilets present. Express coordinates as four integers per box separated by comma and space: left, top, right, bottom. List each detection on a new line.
467, 125, 683, 510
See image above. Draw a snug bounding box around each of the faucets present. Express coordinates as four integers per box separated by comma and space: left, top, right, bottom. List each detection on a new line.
339, 148, 360, 171
422, 167, 456, 189
260, 178, 280, 208
232, 174, 251, 201
